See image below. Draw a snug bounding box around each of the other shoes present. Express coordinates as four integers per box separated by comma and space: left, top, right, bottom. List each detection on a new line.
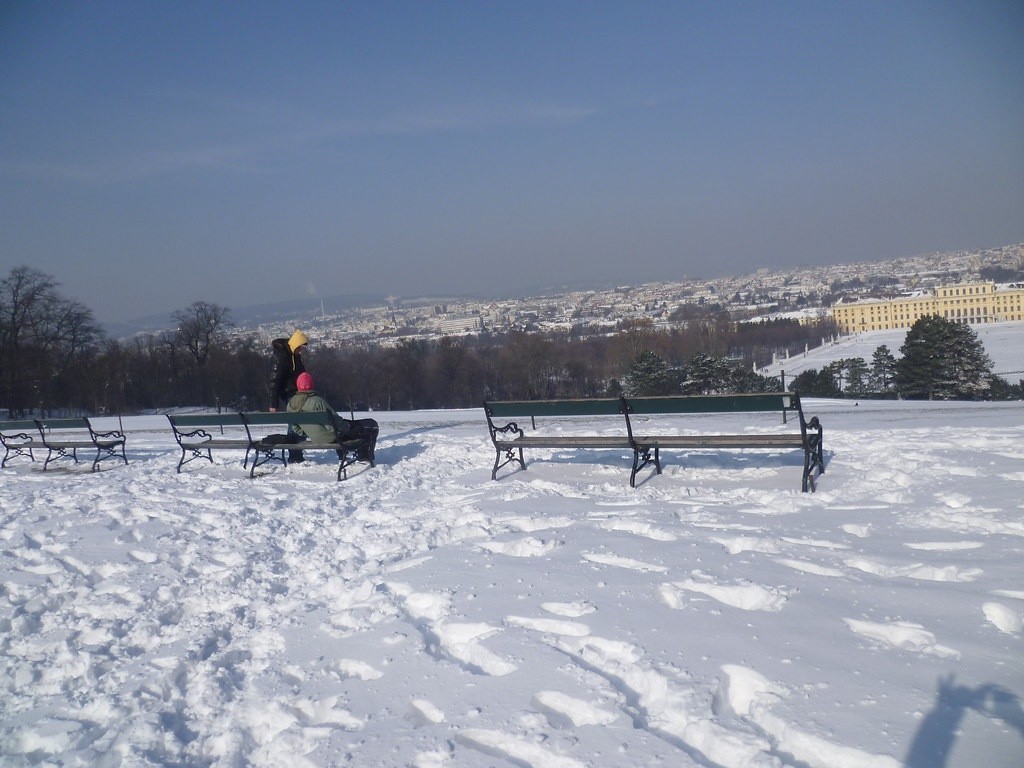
339, 460, 348, 466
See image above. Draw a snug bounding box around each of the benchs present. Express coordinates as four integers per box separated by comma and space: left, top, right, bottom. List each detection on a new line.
0, 416, 128, 473
481, 391, 826, 494
165, 407, 375, 482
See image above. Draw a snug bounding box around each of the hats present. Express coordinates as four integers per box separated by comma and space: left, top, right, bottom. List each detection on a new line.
297, 373, 314, 391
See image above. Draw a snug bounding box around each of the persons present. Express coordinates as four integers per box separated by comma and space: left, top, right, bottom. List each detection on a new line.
286, 372, 379, 466
269, 329, 310, 464
